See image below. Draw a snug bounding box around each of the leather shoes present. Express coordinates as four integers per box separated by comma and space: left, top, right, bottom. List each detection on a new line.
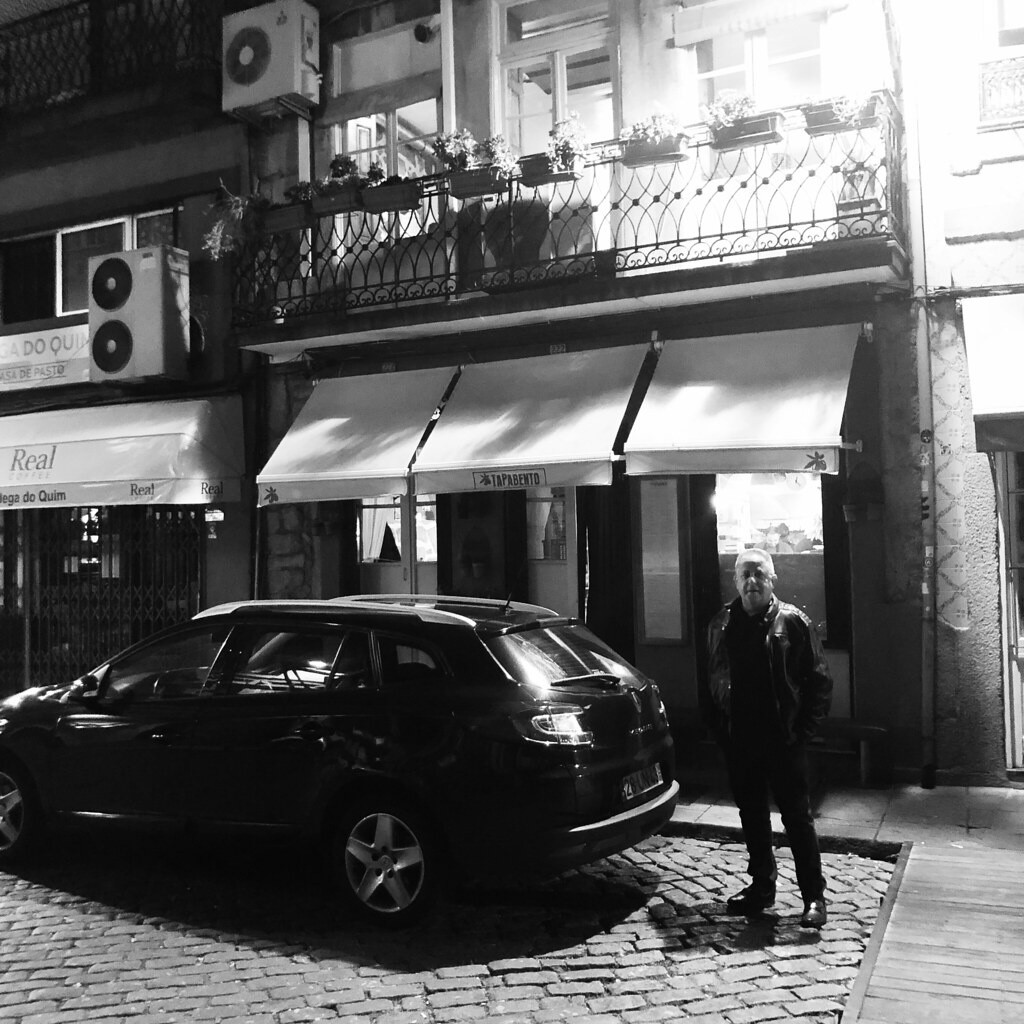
802, 898, 826, 928
727, 887, 776, 906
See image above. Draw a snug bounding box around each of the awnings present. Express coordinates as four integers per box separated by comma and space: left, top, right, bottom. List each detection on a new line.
0, 393, 245, 511
256, 321, 867, 511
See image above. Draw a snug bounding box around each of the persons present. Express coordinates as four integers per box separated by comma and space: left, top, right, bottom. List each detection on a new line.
704, 548, 834, 925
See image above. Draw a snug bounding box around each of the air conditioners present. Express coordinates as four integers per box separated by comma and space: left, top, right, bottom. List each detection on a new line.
222, 0, 319, 134
87, 243, 189, 386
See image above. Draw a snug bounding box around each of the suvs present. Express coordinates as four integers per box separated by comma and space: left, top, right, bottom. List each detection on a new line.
0, 594, 679, 930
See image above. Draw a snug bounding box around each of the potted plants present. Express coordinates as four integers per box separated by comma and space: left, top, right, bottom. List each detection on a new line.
704, 94, 785, 153
432, 128, 509, 199
619, 110, 689, 169
803, 84, 884, 137
362, 174, 423, 214
546, 111, 589, 178
312, 154, 366, 217
248, 182, 314, 233
